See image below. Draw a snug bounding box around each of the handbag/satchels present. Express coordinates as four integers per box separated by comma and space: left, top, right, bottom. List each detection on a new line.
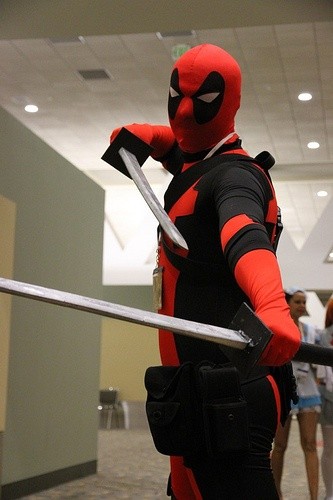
144, 364, 280, 459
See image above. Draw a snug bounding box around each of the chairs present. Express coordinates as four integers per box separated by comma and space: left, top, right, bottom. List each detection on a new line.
100, 390, 118, 428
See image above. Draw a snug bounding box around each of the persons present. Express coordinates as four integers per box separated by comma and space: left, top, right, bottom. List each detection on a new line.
271, 287, 322, 500
110, 43, 301, 500
316, 294, 333, 500
